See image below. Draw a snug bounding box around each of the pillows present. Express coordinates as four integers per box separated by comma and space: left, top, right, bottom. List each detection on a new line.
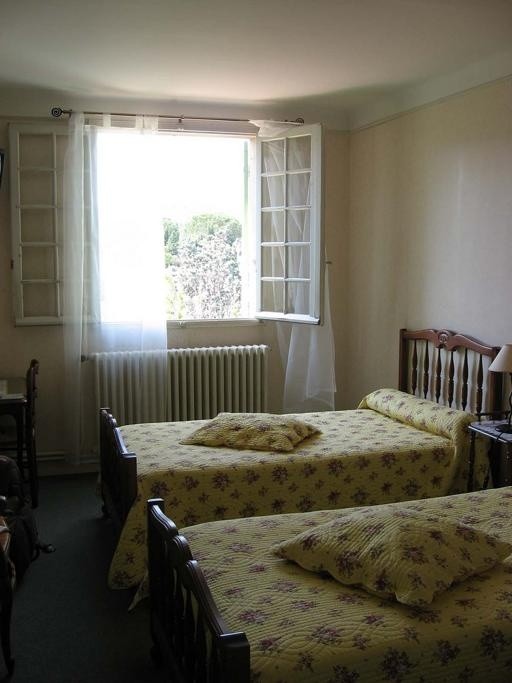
355, 389, 476, 442
269, 506, 509, 607
178, 408, 321, 453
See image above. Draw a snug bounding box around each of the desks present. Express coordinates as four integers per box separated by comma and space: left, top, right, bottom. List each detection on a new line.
467, 416, 511, 490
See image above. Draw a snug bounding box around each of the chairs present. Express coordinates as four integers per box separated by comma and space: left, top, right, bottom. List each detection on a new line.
2, 359, 40, 504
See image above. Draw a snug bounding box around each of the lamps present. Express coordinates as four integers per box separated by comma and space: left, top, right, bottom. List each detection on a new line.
487, 345, 512, 433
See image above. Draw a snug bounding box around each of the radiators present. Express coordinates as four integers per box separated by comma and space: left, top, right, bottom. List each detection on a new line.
77, 344, 271, 462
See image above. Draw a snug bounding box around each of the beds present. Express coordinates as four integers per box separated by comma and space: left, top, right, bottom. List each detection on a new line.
101, 329, 500, 612
149, 484, 512, 680
